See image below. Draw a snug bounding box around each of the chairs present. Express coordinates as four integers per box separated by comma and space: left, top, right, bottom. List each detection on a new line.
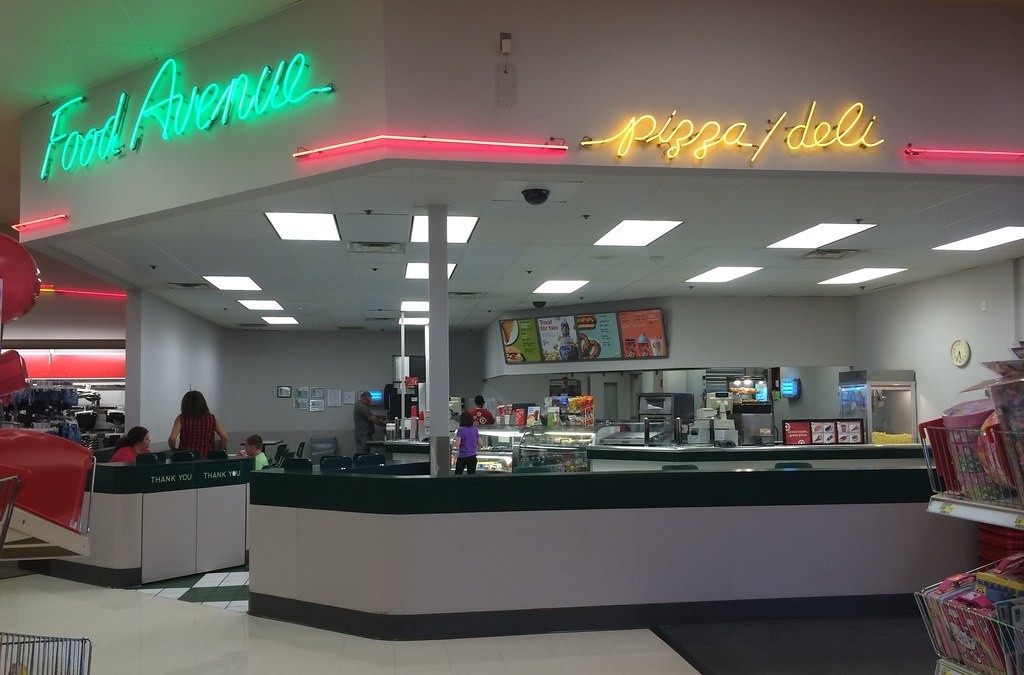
262, 441, 305, 470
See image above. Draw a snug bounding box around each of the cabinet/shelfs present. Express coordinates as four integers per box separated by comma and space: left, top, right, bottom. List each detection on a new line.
914, 418, 1024, 675
726, 375, 771, 414
449, 424, 621, 475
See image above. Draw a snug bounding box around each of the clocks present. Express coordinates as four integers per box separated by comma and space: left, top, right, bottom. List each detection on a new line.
950, 339, 971, 367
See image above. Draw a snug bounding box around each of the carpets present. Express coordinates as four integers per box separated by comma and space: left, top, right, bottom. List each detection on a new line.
646, 612, 942, 675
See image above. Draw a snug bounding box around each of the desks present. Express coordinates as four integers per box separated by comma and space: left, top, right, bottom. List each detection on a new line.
240, 440, 283, 453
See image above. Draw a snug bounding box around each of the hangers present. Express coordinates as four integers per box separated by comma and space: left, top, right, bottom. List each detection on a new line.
74, 406, 125, 417
105, 428, 123, 438
31, 422, 59, 433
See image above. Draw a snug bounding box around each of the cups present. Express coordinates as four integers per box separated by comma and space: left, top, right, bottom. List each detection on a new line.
505, 415, 509, 425
236, 450, 247, 457
625, 340, 636, 357
650, 338, 662, 356
547, 412, 553, 426
638, 341, 649, 357
495, 417, 500, 424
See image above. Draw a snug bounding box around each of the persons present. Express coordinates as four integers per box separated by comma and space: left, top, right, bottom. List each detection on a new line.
245, 435, 269, 471
108, 426, 151, 463
455, 411, 483, 474
353, 391, 389, 453
168, 390, 228, 457
467, 395, 495, 426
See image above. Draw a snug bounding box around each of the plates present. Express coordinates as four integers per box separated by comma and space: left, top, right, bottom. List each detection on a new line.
503, 320, 519, 345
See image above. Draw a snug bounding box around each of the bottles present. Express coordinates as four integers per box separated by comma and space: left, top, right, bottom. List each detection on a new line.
520, 454, 589, 472
449, 439, 458, 470
558, 318, 578, 361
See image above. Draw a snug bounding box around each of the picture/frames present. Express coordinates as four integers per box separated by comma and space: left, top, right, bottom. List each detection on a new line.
276, 385, 325, 411
